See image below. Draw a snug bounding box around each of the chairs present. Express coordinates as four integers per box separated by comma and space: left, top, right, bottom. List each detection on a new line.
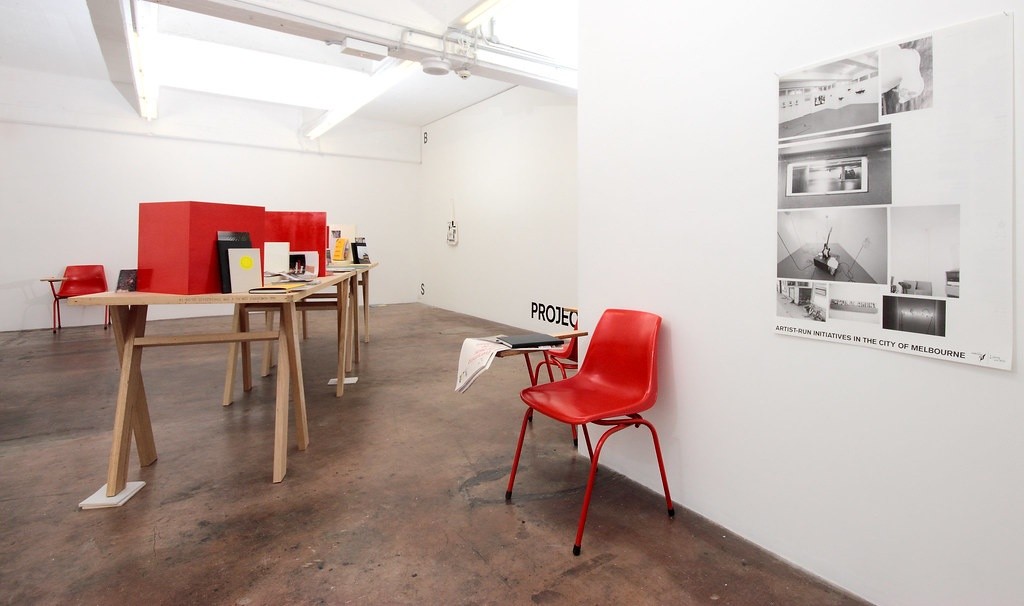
41, 264, 111, 333
506, 309, 674, 555
528, 318, 579, 445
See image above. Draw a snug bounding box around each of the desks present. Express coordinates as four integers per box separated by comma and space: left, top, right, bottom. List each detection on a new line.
495, 329, 588, 388
66, 260, 379, 496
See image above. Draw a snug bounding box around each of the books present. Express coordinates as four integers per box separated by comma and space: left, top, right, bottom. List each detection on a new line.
217, 239, 262, 293
263, 242, 289, 276
249, 283, 305, 294
326, 265, 355, 271
496, 334, 564, 348
